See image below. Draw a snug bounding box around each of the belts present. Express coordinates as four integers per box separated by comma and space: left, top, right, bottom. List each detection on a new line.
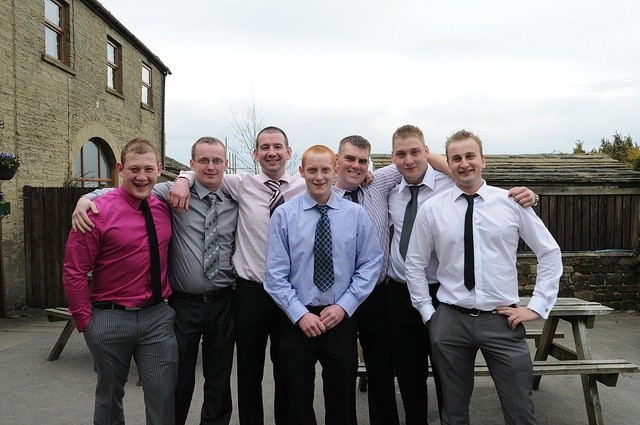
444, 303, 515, 317
171, 292, 230, 303
94, 301, 161, 312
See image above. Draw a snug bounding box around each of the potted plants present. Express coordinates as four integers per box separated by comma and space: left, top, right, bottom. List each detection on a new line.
0, 153, 22, 179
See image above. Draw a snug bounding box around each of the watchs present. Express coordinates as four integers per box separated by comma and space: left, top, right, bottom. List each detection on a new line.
533, 194, 540, 207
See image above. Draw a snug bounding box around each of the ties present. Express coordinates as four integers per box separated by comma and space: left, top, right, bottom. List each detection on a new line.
139, 199, 162, 305
461, 194, 480, 291
313, 205, 335, 291
344, 186, 362, 204
399, 185, 426, 261
203, 194, 220, 280
267, 181, 285, 218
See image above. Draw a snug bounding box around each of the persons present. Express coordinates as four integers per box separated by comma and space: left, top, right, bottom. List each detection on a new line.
331, 135, 456, 425
71, 137, 239, 425
62, 138, 179, 425
263, 145, 385, 425
404, 129, 563, 423
166, 126, 373, 424
386, 125, 539, 425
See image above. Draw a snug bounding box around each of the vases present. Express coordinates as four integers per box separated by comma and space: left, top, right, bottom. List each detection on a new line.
0, 191, 12, 215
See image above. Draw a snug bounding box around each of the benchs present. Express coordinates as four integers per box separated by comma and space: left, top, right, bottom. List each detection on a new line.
525, 329, 565, 339
46, 306, 141, 386
357, 359, 640, 425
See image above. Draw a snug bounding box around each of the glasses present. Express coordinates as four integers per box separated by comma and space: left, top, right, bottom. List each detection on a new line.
194, 158, 225, 165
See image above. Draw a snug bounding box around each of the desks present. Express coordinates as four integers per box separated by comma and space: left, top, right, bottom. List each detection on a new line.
358, 293, 619, 425
